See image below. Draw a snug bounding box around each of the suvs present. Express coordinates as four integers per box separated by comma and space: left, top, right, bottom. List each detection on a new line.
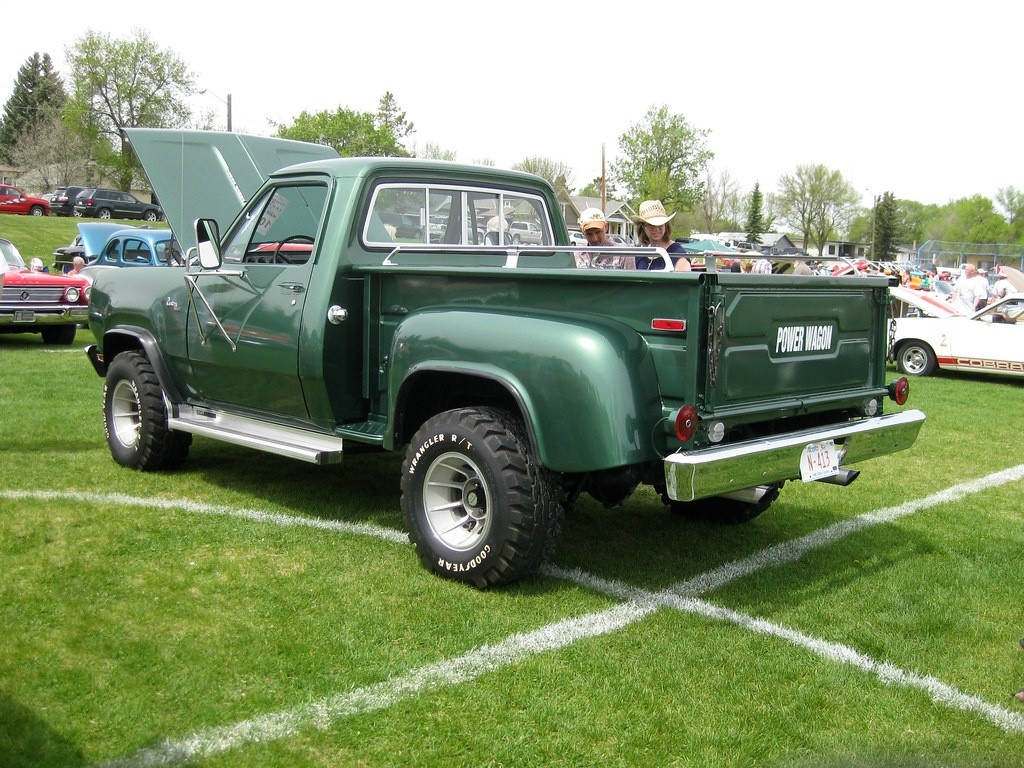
42, 186, 99, 217
71, 188, 165, 222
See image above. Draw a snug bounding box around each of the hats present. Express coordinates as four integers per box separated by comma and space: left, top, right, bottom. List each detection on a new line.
580, 208, 605, 232
630, 199, 677, 226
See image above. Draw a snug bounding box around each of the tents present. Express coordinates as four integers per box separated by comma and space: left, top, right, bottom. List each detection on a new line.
682, 239, 735, 253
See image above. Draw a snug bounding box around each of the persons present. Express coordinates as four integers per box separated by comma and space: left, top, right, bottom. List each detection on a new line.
883, 267, 910, 285
630, 200, 691, 271
487, 216, 509, 233
68, 257, 85, 276
947, 264, 1018, 323
793, 252, 824, 276
723, 258, 772, 274
573, 208, 635, 270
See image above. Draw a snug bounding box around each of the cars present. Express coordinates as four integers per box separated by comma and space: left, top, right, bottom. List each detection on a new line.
51, 223, 183, 273
0, 183, 51, 217
395, 221, 1024, 377
0, 238, 92, 346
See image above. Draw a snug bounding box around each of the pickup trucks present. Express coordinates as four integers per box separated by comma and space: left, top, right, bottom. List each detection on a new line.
84, 126, 928, 593
